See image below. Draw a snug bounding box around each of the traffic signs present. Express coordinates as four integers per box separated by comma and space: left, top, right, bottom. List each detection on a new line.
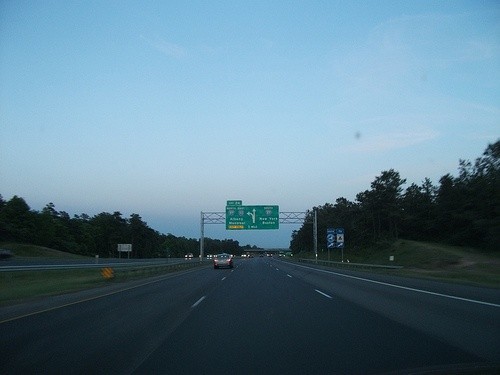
225, 206, 279, 229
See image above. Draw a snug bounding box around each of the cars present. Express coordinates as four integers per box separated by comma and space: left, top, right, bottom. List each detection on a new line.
214, 253, 233, 269
185, 253, 292, 257
